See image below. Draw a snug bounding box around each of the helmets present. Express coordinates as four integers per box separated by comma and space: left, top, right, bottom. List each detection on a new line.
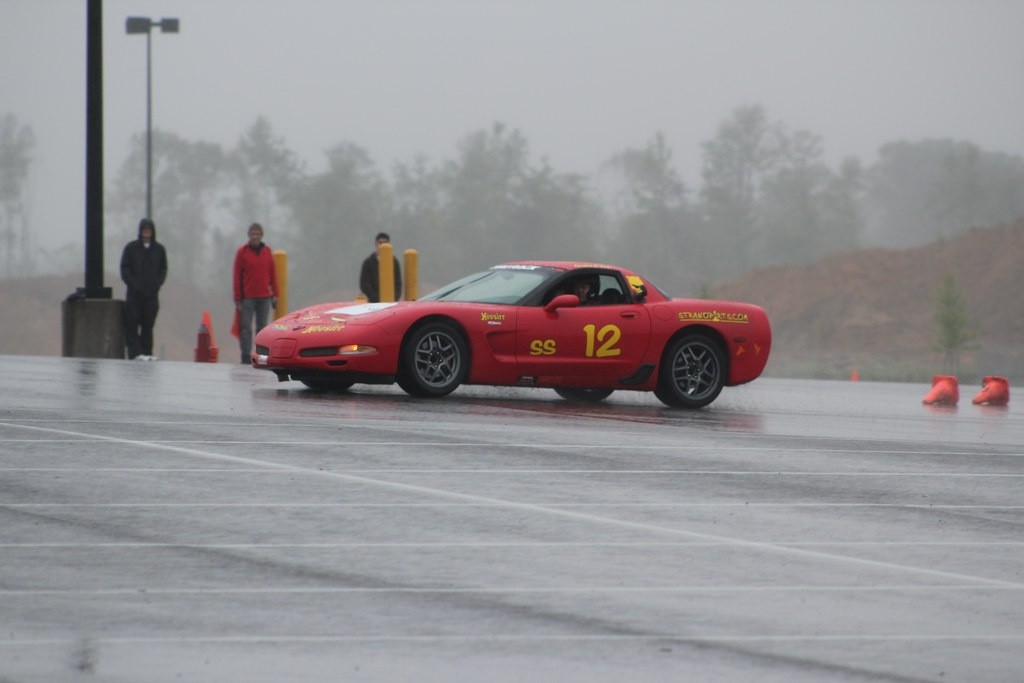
573, 275, 600, 299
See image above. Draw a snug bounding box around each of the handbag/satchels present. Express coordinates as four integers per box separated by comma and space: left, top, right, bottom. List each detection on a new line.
230, 308, 240, 338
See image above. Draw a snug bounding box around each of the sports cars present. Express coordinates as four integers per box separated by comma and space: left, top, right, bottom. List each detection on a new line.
252, 259, 773, 410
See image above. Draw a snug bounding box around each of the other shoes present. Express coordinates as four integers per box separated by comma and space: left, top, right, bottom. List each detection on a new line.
241, 352, 252, 364
135, 354, 158, 361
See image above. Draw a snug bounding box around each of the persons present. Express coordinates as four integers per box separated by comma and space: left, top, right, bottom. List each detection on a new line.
120, 217, 167, 360
573, 273, 601, 306
359, 232, 402, 303
232, 223, 280, 365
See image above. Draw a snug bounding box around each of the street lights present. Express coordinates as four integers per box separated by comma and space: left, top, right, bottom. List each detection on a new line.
122, 16, 179, 234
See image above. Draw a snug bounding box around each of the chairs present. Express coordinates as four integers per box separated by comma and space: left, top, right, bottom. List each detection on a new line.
600, 288, 622, 304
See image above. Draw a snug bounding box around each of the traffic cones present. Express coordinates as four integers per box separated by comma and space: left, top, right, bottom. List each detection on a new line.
922, 376, 961, 407
194, 311, 219, 363
971, 374, 1010, 406
